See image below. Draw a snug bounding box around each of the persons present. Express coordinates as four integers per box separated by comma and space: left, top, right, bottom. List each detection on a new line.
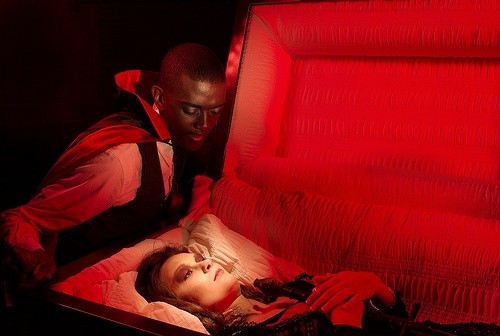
0, 43, 228, 309
135, 242, 500, 336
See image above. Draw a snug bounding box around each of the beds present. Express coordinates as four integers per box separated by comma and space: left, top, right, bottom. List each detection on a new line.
38, 0, 500, 335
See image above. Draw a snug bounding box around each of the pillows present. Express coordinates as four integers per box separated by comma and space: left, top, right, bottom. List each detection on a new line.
82, 210, 312, 334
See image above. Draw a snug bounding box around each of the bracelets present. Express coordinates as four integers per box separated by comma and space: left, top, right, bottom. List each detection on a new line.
388, 286, 397, 309
